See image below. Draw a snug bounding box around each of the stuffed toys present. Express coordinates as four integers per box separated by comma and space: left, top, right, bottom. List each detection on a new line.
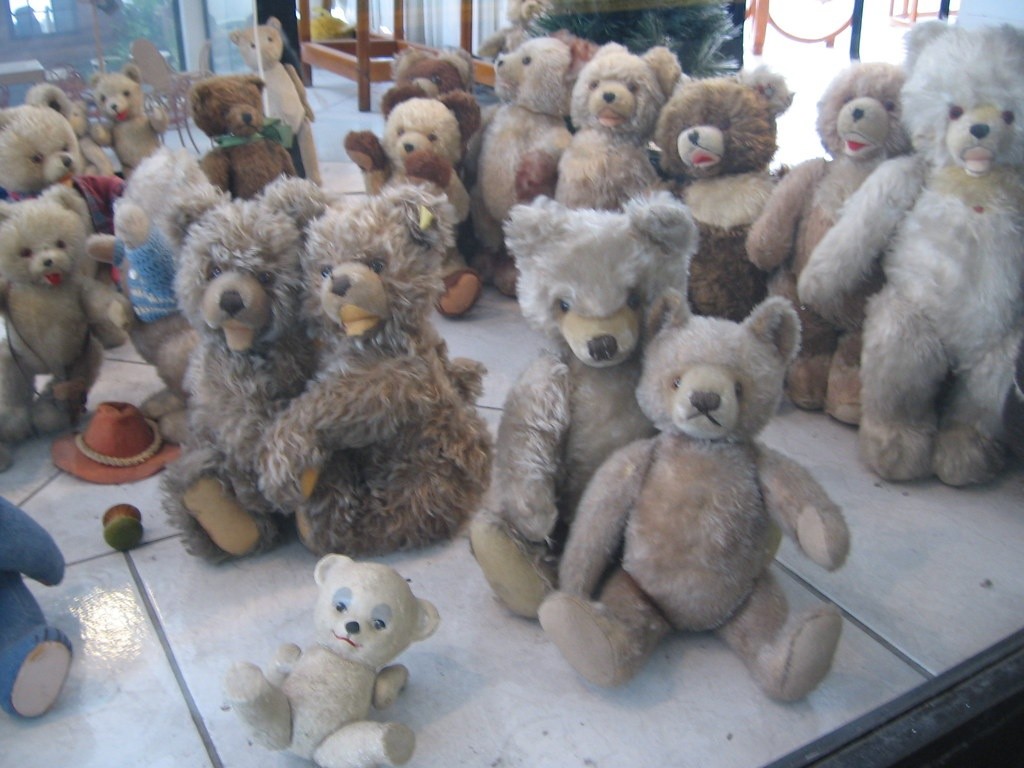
1, 0, 1024, 725
223, 553, 440, 768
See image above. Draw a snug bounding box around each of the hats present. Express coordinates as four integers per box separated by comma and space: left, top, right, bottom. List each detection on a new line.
50, 402, 182, 486
